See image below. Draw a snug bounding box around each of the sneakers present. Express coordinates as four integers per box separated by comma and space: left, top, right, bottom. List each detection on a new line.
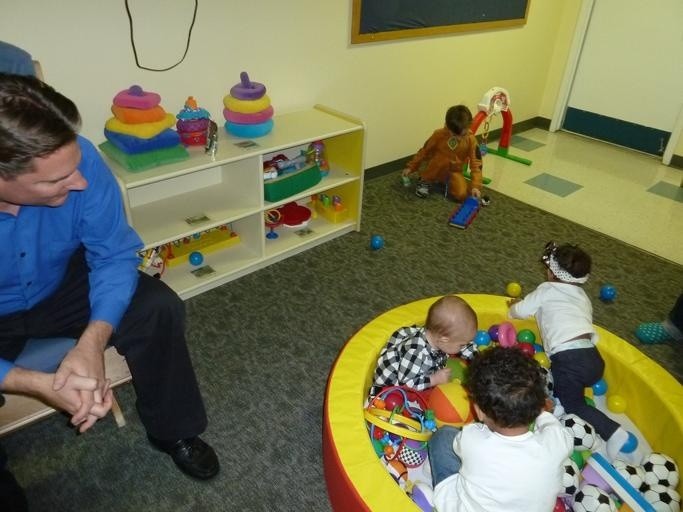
415, 176, 431, 199
636, 322, 673, 345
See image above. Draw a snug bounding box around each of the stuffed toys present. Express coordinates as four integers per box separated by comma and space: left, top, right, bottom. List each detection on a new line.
222, 73, 274, 138
175, 95, 209, 146
97, 85, 189, 173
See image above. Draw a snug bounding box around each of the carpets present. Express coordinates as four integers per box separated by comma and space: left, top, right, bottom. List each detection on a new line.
0, 156, 683, 512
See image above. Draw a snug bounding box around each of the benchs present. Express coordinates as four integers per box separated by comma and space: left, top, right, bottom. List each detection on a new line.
0, 347, 133, 436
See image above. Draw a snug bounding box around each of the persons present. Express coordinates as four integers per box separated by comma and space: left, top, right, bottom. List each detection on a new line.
0, 72, 222, 512
373, 295, 480, 395
635, 289, 683, 345
427, 346, 574, 511
505, 242, 629, 462
399, 105, 483, 203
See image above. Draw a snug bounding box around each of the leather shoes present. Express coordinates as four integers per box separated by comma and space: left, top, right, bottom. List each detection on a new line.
164, 436, 220, 480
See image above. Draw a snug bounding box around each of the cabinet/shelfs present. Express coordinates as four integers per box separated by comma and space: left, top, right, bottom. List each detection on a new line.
95, 105, 365, 301
